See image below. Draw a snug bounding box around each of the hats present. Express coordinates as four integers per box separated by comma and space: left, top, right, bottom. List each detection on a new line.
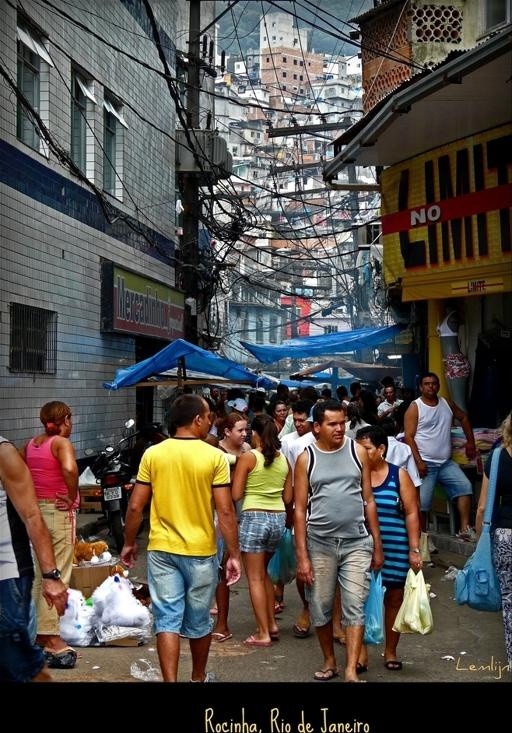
228, 398, 249, 412
305, 404, 316, 423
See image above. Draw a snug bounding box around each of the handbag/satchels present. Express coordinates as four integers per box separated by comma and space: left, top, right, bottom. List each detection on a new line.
419, 532, 431, 563
453, 532, 501, 612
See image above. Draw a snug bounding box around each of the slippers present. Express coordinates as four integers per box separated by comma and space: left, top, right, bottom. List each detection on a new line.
314, 629, 403, 681
210, 596, 309, 647
43, 645, 82, 659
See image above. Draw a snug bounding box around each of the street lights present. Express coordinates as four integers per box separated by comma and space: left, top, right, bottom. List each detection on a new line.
274, 246, 373, 366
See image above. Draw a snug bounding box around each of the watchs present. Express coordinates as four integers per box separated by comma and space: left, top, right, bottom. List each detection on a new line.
43, 568, 61, 580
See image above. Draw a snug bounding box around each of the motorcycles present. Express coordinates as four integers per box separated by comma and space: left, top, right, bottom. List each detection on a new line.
83, 417, 144, 552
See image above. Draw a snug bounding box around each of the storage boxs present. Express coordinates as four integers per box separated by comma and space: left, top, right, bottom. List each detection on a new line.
69, 565, 115, 602
79, 485, 105, 515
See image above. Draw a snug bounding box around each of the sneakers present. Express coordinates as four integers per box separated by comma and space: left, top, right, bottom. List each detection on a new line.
190, 672, 215, 683
456, 528, 478, 542
428, 542, 438, 554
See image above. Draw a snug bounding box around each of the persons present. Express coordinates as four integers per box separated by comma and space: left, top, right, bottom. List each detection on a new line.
18, 401, 82, 659
120, 373, 512, 683
0, 436, 68, 684
